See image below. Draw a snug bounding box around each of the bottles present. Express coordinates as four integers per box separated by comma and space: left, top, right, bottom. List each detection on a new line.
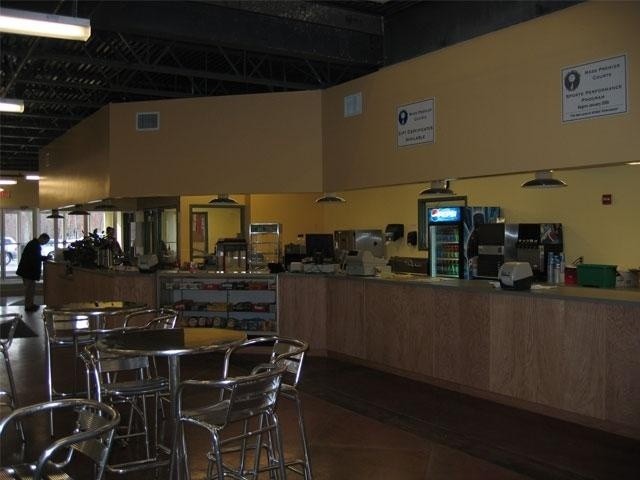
435, 226, 461, 276
189, 261, 198, 274
295, 234, 307, 254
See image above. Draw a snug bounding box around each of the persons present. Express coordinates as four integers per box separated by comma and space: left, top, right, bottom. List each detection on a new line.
15, 231, 52, 312
103, 226, 120, 253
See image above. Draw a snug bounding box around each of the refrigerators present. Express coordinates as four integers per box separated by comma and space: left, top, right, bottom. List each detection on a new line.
427, 206, 501, 281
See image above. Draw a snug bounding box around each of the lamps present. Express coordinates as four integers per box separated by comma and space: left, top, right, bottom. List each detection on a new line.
47, 199, 119, 219
314, 193, 346, 204
0, 98, 25, 114
522, 170, 567, 190
0, 8, 93, 42
208, 193, 239, 206
418, 181, 454, 197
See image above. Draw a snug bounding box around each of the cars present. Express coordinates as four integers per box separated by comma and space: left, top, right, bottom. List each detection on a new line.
3, 236, 102, 266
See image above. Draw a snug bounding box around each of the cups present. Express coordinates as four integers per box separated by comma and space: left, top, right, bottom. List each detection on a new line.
546, 250, 566, 284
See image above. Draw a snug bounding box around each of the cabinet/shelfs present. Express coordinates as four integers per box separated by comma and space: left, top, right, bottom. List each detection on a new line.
248, 223, 282, 270
157, 270, 281, 354
217, 239, 247, 271
